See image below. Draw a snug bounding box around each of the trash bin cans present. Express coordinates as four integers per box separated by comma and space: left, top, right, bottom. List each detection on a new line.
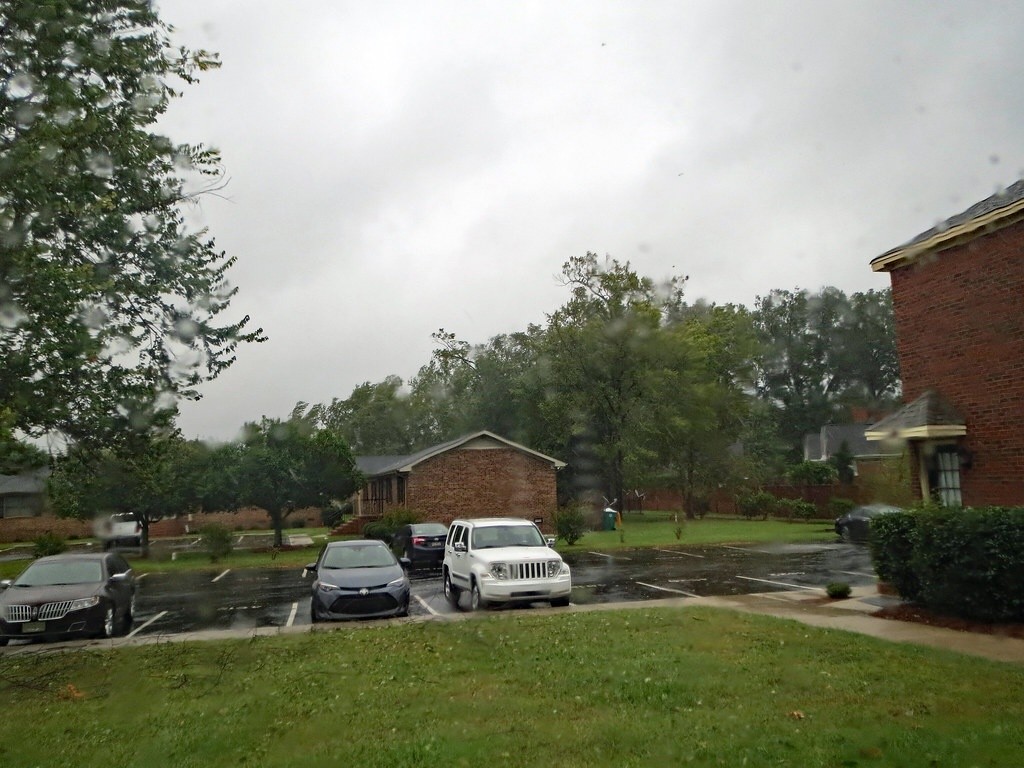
604, 511, 618, 530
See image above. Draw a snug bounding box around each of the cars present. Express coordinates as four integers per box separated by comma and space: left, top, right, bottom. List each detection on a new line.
835, 504, 904, 542
0, 548, 137, 647
388, 523, 449, 575
305, 539, 412, 624
102, 511, 144, 548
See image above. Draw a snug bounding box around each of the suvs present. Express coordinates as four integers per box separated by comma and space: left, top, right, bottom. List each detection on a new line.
442, 517, 572, 613
